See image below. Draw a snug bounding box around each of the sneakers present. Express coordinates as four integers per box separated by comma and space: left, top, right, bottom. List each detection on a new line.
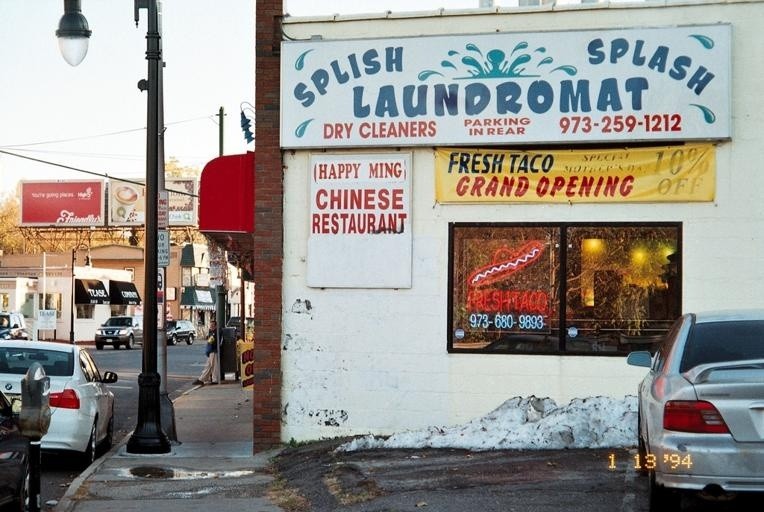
209, 381, 218, 384
192, 380, 204, 385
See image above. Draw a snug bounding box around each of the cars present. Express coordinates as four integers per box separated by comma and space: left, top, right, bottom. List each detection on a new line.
95, 315, 143, 350
166, 319, 198, 345
627, 313, 764, 511
482, 334, 580, 354
227, 316, 254, 327
0, 311, 118, 512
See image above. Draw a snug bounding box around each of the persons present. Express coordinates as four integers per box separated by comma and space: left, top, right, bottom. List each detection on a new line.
191, 321, 217, 385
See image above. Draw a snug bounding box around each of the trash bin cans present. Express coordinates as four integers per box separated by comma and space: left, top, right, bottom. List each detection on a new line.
221, 327, 242, 381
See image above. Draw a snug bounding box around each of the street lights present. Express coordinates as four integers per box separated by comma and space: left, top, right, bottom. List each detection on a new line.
55, 0, 171, 453
70, 243, 92, 343
